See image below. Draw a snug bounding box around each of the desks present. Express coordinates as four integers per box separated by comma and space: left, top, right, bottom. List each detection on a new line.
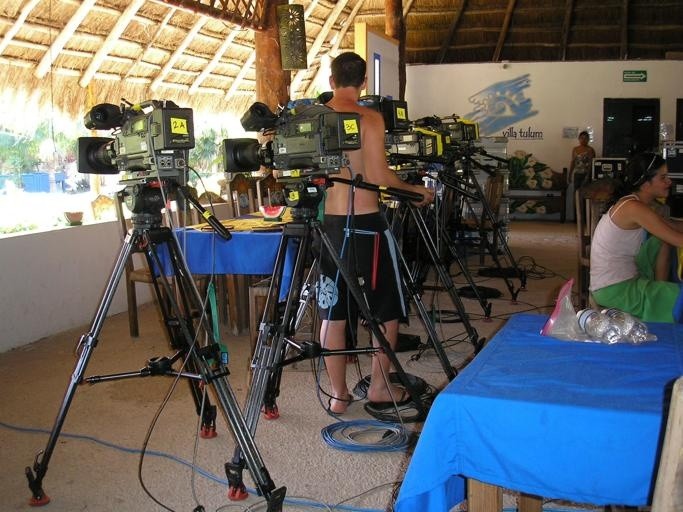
432, 308, 683, 511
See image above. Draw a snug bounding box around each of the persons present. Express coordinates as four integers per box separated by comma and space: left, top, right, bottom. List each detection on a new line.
297, 91, 359, 365
310, 49, 439, 415
586, 151, 682, 325
566, 129, 595, 224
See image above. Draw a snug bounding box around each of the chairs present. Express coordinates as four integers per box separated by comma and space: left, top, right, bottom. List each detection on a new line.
110, 173, 330, 369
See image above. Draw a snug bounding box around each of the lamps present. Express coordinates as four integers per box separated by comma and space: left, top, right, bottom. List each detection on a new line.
276, 3, 308, 72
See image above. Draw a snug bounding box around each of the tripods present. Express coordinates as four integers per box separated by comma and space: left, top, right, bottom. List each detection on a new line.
23, 214, 287, 512
225, 221, 430, 501
344, 165, 529, 384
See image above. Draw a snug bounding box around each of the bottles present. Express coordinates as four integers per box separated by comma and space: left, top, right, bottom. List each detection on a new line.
577, 304, 620, 348
600, 299, 651, 344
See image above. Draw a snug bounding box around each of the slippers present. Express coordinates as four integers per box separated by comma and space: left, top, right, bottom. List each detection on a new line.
327, 394, 353, 415
370, 386, 411, 409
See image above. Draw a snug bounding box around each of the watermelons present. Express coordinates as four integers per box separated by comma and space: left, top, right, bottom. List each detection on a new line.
64, 211, 83, 223
259, 206, 287, 218
